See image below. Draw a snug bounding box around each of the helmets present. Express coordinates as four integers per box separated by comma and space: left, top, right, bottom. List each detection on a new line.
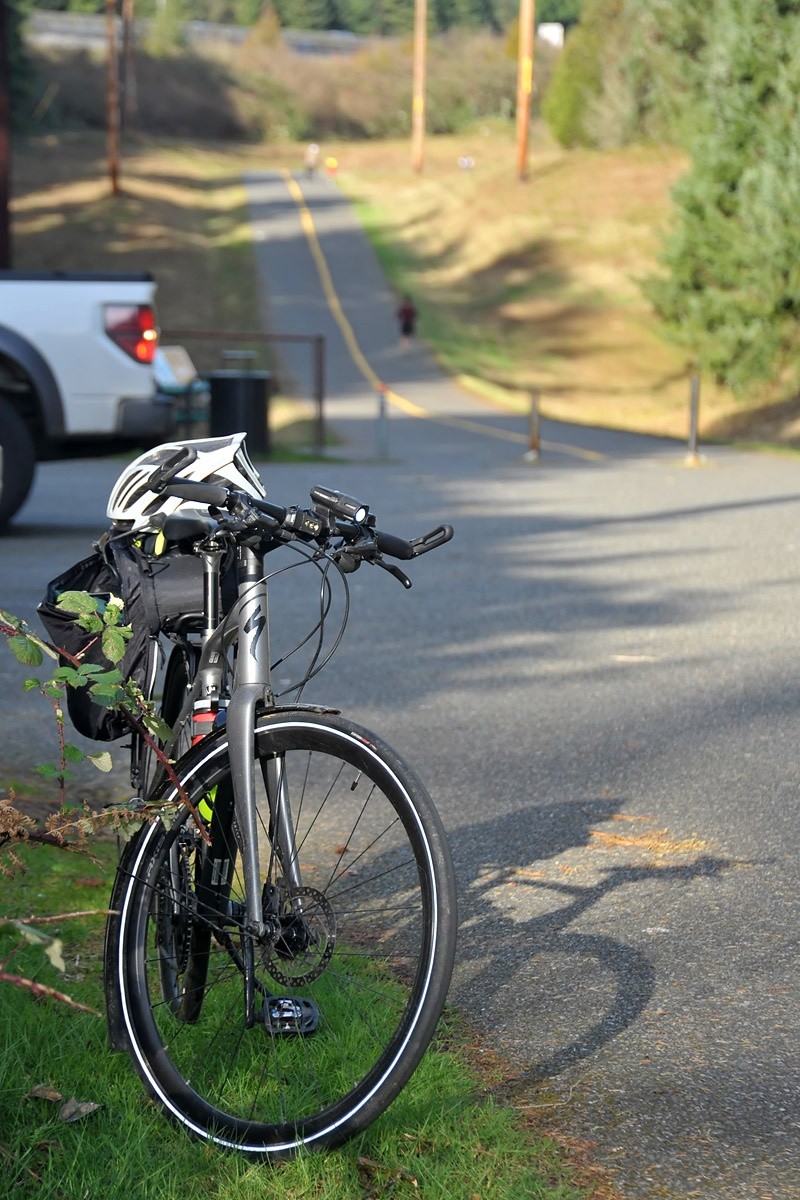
107, 431, 266, 535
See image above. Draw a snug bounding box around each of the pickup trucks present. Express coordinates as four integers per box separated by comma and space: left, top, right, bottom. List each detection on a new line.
1, 265, 187, 534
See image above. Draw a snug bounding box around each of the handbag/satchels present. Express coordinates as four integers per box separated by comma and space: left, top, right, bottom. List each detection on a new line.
35, 549, 162, 741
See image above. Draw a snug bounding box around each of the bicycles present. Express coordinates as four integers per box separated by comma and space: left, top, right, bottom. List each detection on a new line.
97, 471, 469, 1160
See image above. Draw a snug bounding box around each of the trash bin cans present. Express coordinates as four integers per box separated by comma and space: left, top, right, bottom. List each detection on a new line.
207, 350, 269, 457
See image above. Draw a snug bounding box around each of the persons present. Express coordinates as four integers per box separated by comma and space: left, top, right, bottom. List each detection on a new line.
399, 296, 417, 347
307, 145, 319, 178
